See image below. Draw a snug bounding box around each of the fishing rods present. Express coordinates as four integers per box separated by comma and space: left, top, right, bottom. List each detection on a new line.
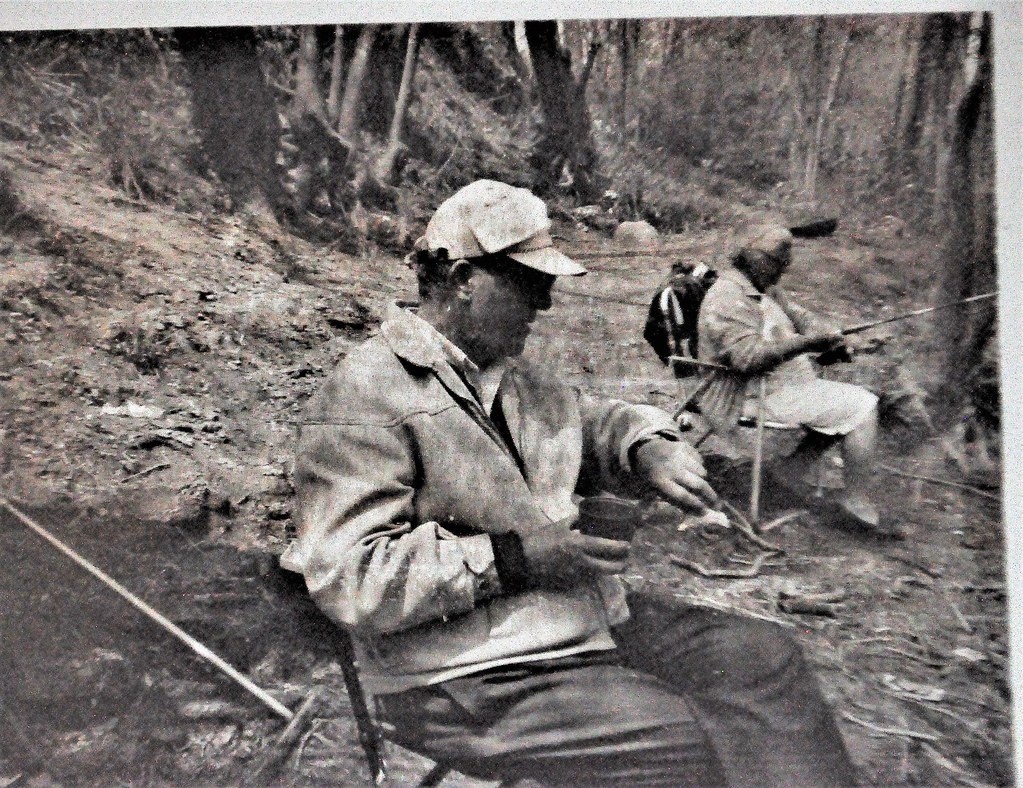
837, 289, 1000, 335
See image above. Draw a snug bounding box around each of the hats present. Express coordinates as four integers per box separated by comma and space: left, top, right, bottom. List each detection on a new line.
426, 178, 588, 276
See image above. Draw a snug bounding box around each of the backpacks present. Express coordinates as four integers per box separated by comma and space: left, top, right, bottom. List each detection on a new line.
644, 260, 705, 376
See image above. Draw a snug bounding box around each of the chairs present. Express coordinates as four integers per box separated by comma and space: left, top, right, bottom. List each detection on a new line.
257, 553, 528, 788
659, 286, 810, 534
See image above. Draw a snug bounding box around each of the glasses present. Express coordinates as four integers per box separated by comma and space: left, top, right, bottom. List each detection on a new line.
468, 254, 559, 297
759, 248, 789, 269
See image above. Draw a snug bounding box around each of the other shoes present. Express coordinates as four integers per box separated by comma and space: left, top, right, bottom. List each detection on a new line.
830, 489, 908, 540
767, 458, 836, 511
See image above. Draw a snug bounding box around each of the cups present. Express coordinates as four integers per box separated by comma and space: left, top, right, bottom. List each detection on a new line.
578, 498, 639, 560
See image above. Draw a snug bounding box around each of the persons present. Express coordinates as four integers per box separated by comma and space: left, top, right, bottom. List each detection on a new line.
294, 178, 860, 788
696, 222, 910, 540
